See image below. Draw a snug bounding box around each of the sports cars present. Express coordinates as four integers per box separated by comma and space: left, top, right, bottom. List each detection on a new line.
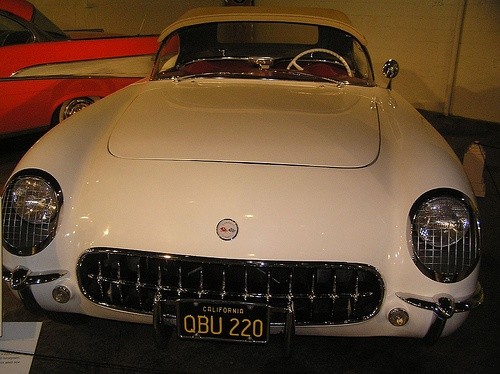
0, 0, 180, 133
1, 5, 483, 353
